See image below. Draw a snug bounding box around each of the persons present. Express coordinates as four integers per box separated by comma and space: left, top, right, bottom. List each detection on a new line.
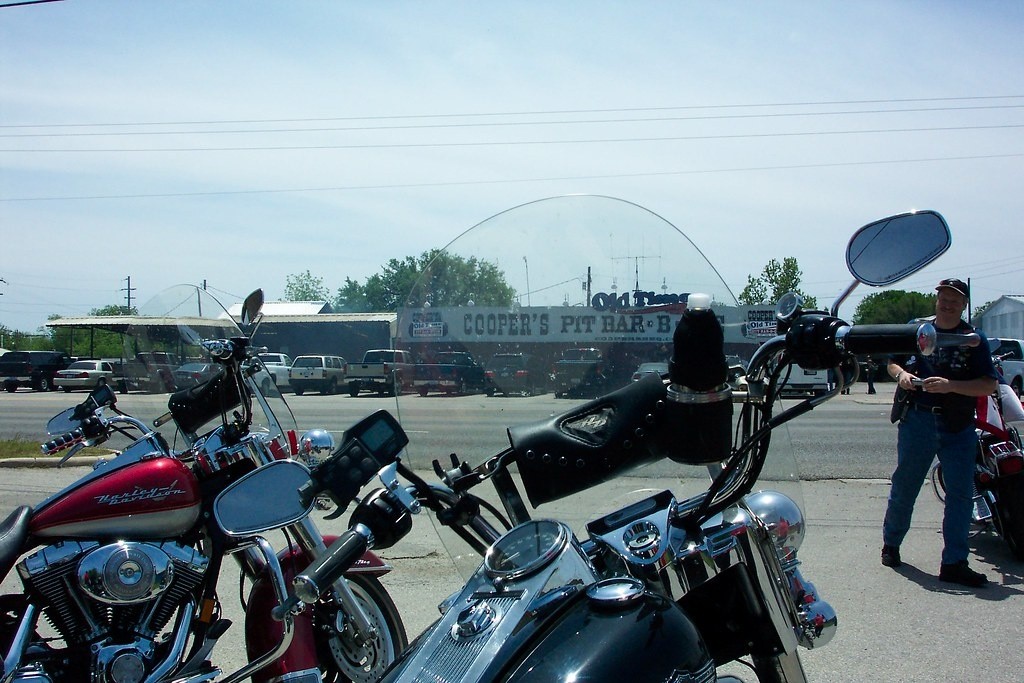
881, 277, 998, 586
839, 355, 876, 396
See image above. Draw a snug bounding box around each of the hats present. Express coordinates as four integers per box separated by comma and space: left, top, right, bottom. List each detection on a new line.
935, 278, 969, 298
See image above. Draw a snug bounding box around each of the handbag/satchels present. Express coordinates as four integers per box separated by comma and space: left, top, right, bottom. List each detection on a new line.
891, 362, 921, 423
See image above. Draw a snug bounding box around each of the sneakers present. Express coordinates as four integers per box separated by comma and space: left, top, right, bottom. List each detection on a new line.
939, 560, 988, 584
881, 544, 901, 567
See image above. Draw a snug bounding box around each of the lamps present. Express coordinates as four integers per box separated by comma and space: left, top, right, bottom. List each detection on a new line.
467, 293, 475, 308
515, 293, 521, 308
562, 293, 570, 307
661, 277, 668, 294
712, 294, 716, 304
424, 294, 432, 309
611, 277, 617, 295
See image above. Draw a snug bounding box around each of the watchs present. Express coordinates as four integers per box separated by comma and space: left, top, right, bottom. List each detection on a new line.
895, 370, 902, 383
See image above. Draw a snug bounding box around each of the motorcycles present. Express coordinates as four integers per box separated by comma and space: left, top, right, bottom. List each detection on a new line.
930, 338, 1024, 566
211, 190, 980, 682
0, 283, 415, 682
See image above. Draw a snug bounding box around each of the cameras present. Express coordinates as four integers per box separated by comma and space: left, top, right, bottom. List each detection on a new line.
912, 378, 928, 391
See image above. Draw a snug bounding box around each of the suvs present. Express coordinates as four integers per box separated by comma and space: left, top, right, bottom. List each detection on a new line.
288, 352, 350, 396
988, 338, 1024, 400
0, 350, 74, 393
768, 349, 828, 399
482, 351, 552, 398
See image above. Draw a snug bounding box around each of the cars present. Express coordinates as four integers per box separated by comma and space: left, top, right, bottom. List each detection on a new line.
170, 362, 222, 394
53, 361, 118, 393
630, 361, 668, 385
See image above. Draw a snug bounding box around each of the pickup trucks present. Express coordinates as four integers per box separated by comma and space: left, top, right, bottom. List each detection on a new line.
552, 347, 613, 400
343, 348, 414, 397
112, 352, 182, 393
410, 351, 491, 397
240, 352, 291, 396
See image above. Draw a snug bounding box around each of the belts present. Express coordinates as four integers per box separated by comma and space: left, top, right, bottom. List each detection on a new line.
910, 401, 947, 415
